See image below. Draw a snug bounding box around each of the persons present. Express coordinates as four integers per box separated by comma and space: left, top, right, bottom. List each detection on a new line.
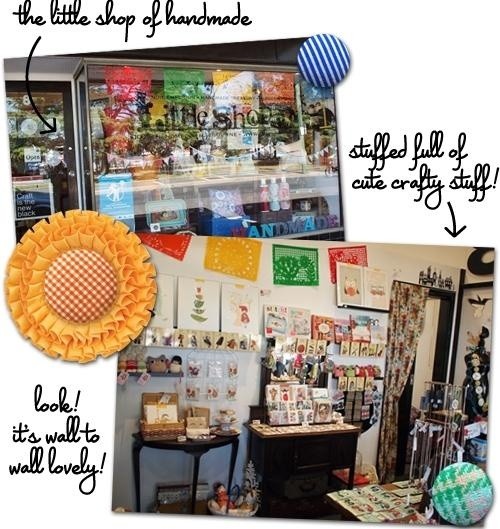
319, 403, 331, 421
305, 103, 318, 118
316, 101, 334, 130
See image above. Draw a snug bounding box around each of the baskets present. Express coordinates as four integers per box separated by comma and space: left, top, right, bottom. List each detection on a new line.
208, 497, 258, 517
140, 417, 185, 441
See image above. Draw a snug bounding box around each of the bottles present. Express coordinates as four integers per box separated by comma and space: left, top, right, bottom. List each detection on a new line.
269, 178, 280, 212
260, 178, 270, 213
280, 176, 291, 213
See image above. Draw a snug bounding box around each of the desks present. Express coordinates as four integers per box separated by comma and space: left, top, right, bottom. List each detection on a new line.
130, 404, 424, 524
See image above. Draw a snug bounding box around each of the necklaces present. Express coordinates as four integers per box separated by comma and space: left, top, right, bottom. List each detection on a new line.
408, 381, 468, 491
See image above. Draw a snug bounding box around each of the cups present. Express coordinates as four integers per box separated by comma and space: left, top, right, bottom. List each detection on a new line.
298, 200, 312, 212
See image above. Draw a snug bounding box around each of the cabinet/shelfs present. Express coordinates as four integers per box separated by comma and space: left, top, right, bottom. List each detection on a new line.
93, 171, 343, 240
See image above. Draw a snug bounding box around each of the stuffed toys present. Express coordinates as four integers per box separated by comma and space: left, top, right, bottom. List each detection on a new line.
212, 479, 227, 510
244, 489, 257, 513
169, 355, 183, 373
147, 354, 170, 373
228, 484, 241, 509
137, 351, 147, 373
119, 353, 127, 372
127, 350, 137, 372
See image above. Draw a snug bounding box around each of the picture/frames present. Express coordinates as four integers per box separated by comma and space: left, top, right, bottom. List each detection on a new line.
336, 261, 390, 309
